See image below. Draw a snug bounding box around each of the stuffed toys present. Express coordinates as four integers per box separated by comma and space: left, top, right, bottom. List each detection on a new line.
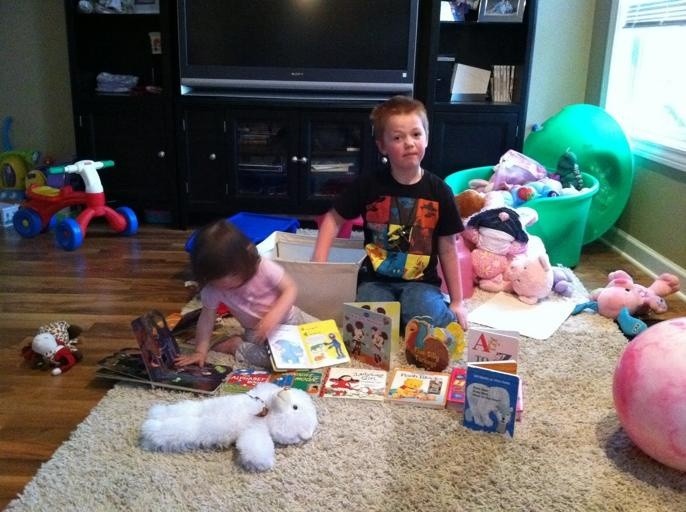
21, 321, 83, 377
456, 145, 591, 217
571, 269, 680, 337
461, 203, 554, 305
552, 267, 573, 299
137, 379, 318, 474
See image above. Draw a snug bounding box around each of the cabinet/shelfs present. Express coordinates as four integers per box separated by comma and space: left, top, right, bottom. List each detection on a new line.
61, 0, 541, 231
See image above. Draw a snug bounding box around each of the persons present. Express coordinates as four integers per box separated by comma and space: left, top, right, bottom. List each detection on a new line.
173, 219, 320, 368
309, 94, 469, 338
453, 0, 514, 17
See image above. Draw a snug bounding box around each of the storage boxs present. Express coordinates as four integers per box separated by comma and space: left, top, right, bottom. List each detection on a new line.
247, 230, 372, 326
443, 163, 603, 269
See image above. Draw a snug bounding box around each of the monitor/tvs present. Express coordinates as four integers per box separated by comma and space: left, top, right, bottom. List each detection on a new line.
176, 0, 418, 102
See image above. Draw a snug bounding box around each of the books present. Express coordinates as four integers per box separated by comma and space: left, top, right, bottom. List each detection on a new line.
94, 307, 235, 396
404, 317, 465, 372
310, 157, 356, 197
490, 63, 515, 104
387, 371, 450, 409
346, 146, 360, 152
343, 302, 400, 372
237, 117, 281, 146
320, 368, 387, 402
446, 359, 525, 438
468, 327, 520, 362
223, 320, 351, 395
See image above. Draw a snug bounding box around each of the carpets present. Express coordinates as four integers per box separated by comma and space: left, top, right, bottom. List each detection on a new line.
3, 266, 686, 512
